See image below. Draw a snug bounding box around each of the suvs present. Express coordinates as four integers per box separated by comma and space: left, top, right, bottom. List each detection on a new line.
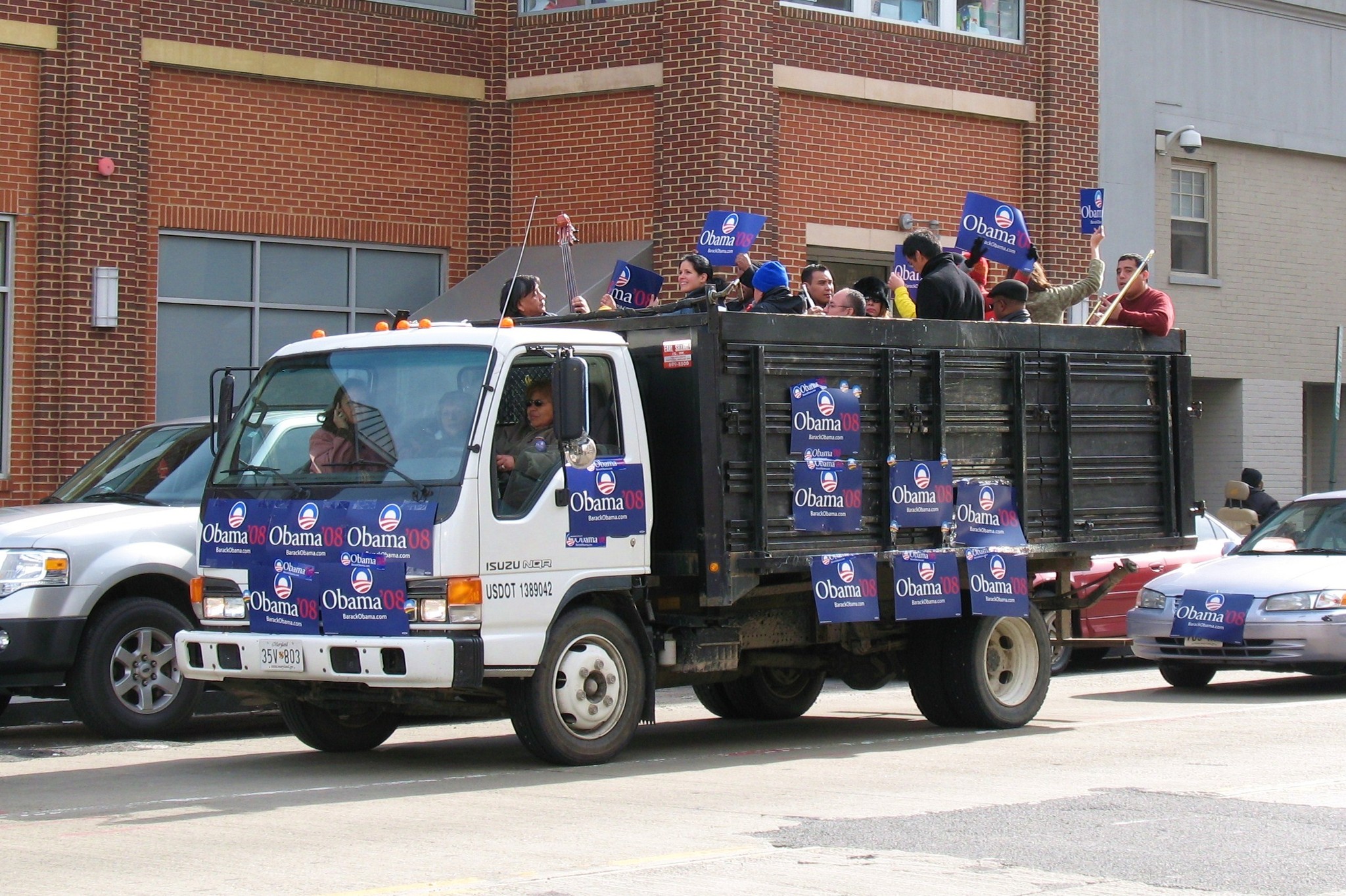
1, 405, 325, 734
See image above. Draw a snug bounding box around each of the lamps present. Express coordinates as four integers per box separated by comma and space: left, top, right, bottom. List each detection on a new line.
91, 266, 119, 328
900, 215, 942, 247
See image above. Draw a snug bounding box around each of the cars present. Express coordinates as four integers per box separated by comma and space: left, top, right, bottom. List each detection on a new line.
1125, 490, 1346, 690
1024, 509, 1298, 680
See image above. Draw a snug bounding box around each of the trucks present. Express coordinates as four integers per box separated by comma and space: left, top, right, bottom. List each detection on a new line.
171, 279, 1201, 770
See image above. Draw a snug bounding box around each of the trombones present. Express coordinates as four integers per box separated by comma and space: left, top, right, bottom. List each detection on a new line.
1081, 248, 1157, 326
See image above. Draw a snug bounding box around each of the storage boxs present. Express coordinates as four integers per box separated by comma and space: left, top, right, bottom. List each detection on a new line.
957, 4, 981, 32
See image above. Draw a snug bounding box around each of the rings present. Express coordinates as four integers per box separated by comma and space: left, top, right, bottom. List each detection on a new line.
501, 465, 506, 471
338, 414, 342, 417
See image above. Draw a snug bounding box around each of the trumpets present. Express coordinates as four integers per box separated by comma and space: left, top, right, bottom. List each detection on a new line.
802, 283, 823, 312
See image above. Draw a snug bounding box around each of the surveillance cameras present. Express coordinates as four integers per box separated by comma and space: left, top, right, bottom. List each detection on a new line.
1179, 129, 1203, 153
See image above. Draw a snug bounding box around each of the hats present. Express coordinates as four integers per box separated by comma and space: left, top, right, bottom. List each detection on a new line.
854, 277, 890, 309
987, 280, 1028, 301
751, 260, 788, 294
1242, 468, 1261, 487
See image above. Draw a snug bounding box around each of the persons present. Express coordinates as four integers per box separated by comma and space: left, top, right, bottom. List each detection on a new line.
308, 378, 598, 513
1099, 253, 1175, 338
1224, 467, 1280, 530
499, 274, 591, 318
726, 251, 891, 318
598, 254, 725, 318
888, 225, 1105, 324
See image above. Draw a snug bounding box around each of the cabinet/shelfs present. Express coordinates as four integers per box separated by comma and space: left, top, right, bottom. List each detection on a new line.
978, 0, 1017, 37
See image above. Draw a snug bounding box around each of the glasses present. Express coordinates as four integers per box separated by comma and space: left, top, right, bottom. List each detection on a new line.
827, 303, 855, 313
864, 295, 882, 302
526, 399, 552, 407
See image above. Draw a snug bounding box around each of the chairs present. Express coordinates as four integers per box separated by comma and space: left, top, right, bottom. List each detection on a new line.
1218, 480, 1261, 535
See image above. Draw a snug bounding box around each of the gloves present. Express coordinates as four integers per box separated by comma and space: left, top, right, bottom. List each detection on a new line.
970, 237, 989, 263
1027, 244, 1037, 259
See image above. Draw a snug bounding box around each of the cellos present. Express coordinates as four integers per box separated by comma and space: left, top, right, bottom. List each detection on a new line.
553, 213, 582, 313
